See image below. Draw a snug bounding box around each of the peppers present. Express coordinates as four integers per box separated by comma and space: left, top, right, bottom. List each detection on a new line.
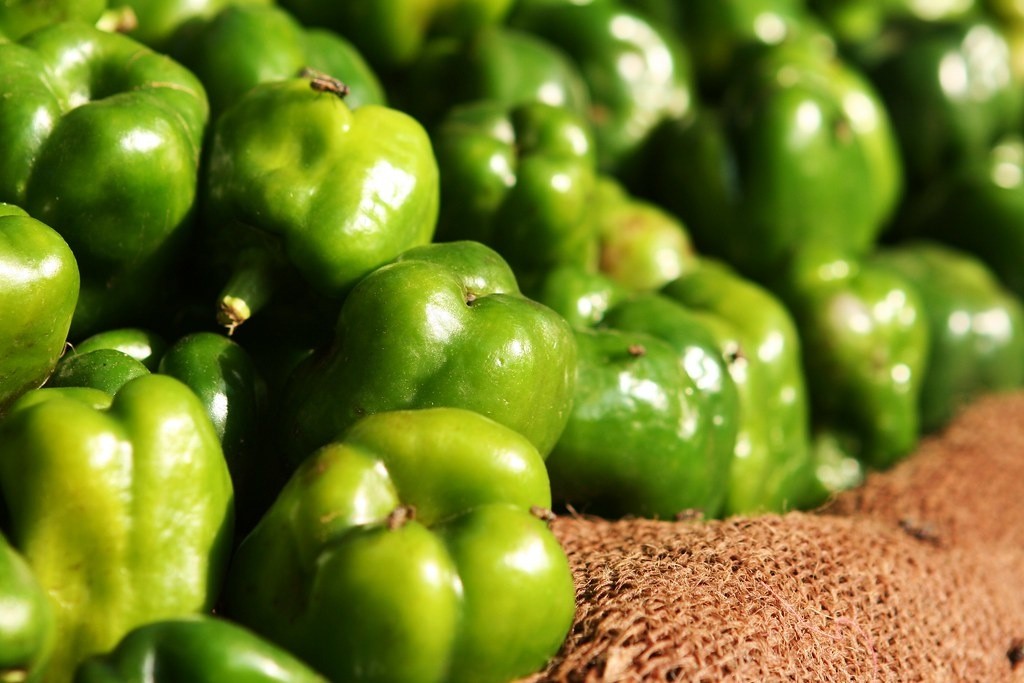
0, 0, 1024, 683
